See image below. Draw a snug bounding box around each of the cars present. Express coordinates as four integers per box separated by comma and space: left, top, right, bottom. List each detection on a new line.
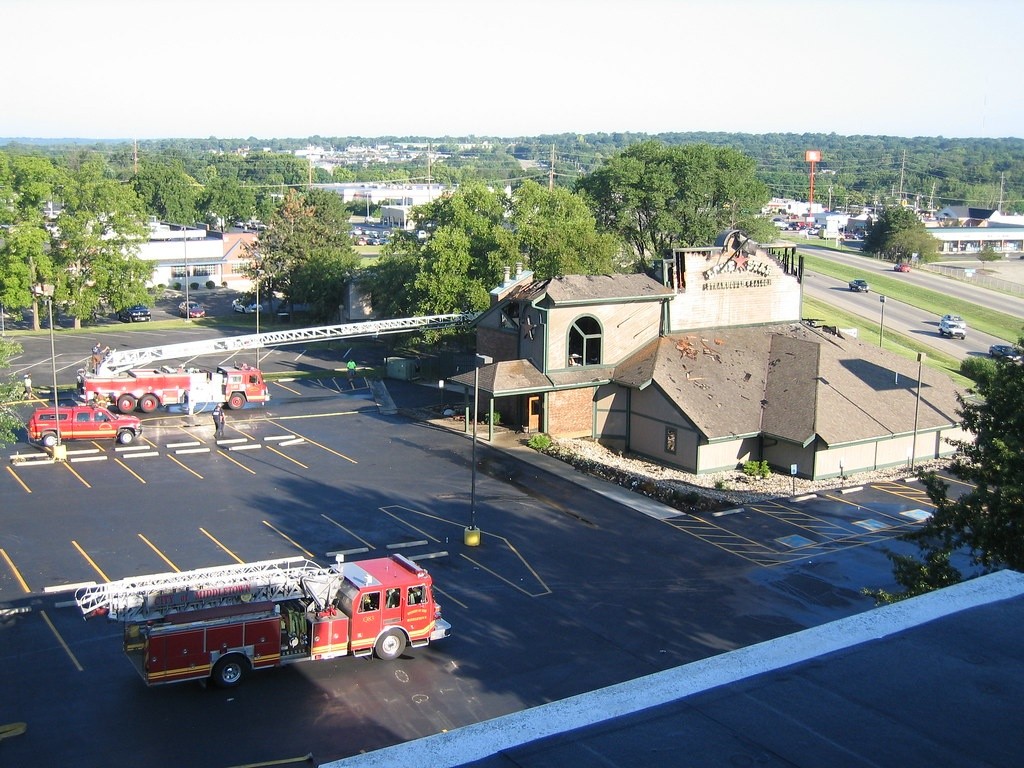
941, 314, 966, 329
232, 298, 263, 313
809, 230, 819, 235
353, 229, 362, 235
178, 301, 206, 318
369, 231, 380, 238
989, 344, 1020, 362
366, 239, 380, 246
379, 238, 390, 245
814, 223, 821, 228
255, 222, 267, 230
116, 305, 151, 322
894, 263, 910, 272
362, 229, 370, 235
358, 237, 367, 245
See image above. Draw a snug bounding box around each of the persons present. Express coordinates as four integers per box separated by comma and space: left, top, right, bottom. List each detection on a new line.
92, 342, 109, 373
347, 358, 356, 383
212, 402, 225, 439
21, 372, 34, 401
89, 392, 116, 413
184, 389, 188, 404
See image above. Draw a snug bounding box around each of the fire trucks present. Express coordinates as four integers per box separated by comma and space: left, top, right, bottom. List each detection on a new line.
72, 312, 476, 415
75, 554, 452, 690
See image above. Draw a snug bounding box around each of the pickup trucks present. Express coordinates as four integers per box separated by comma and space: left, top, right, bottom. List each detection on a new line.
28, 400, 143, 447
849, 279, 869, 293
938, 321, 966, 340
